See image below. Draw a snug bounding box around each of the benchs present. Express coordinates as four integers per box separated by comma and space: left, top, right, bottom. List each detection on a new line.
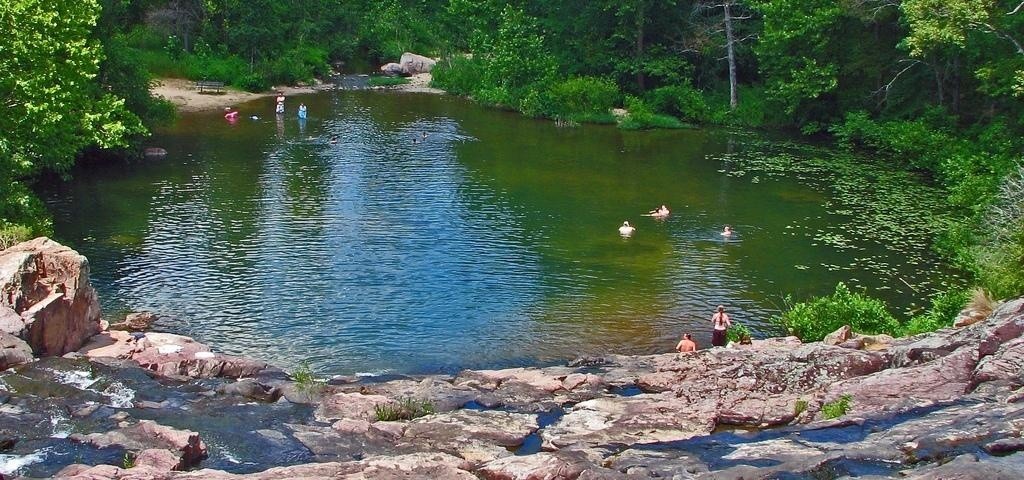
195, 81, 224, 94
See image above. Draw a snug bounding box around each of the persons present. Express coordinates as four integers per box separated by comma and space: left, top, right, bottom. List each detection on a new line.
710, 304, 731, 346
420, 132, 427, 138
330, 138, 337, 144
275, 91, 286, 114
720, 226, 731, 236
674, 333, 695, 354
660, 205, 669, 214
648, 207, 660, 218
297, 103, 306, 120
222, 108, 238, 120
617, 220, 636, 236
411, 138, 420, 144
